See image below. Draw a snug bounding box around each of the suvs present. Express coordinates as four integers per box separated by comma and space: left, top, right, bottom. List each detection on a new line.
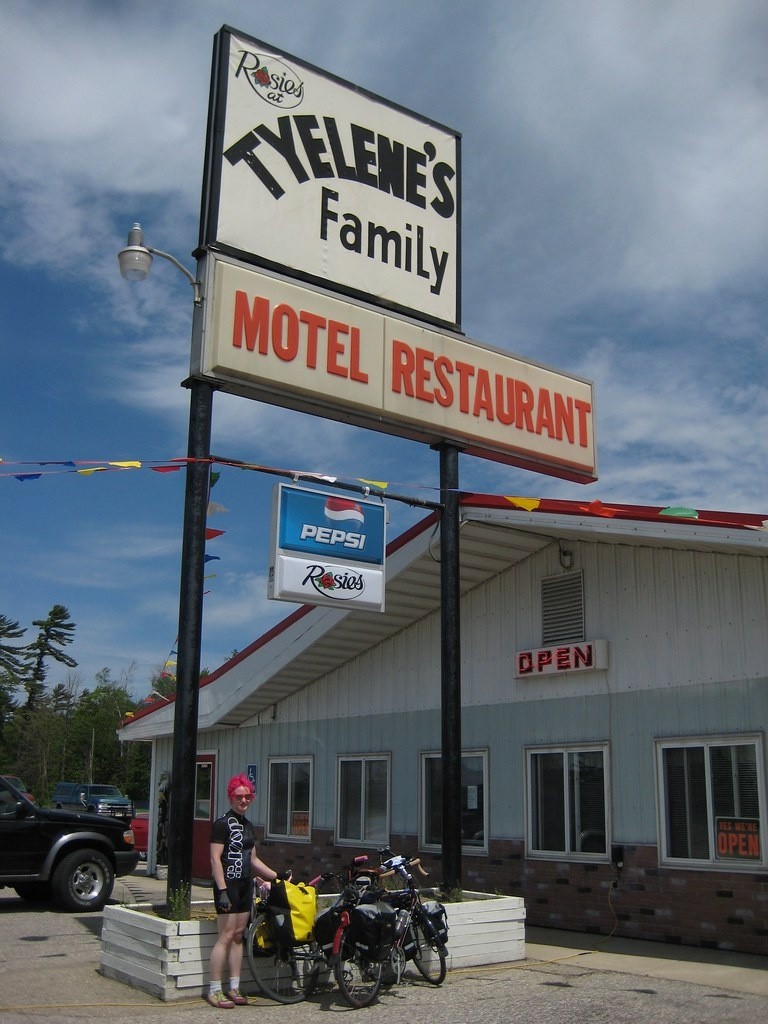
0, 775, 140, 912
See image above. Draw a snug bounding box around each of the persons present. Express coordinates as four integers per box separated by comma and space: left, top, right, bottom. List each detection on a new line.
208, 772, 293, 1008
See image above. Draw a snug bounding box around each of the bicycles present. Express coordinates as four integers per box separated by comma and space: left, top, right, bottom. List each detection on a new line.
244, 844, 450, 1008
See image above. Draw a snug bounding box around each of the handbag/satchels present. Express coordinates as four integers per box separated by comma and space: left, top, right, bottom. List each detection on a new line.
354, 903, 397, 946
268, 879, 317, 945
313, 907, 356, 961
242, 897, 276, 957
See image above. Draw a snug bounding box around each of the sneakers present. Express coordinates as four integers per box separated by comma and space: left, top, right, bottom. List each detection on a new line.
208, 990, 235, 1008
228, 989, 247, 1005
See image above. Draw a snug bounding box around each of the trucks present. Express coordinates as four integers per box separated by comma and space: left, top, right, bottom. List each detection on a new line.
49, 779, 136, 828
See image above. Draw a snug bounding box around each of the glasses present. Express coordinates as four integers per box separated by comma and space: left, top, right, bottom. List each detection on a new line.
232, 794, 252, 801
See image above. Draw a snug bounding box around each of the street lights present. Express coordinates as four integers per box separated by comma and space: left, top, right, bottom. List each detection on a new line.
115, 220, 219, 923
